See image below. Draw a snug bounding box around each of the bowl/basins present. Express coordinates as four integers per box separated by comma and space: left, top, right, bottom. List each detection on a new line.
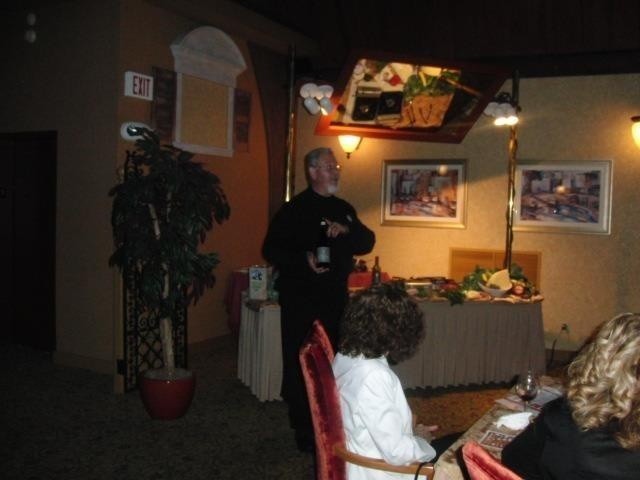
477, 281, 513, 297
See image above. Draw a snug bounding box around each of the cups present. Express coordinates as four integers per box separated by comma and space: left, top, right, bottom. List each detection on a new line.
515, 368, 540, 412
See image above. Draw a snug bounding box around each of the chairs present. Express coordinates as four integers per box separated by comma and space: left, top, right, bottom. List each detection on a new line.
297, 321, 439, 480
459, 436, 534, 480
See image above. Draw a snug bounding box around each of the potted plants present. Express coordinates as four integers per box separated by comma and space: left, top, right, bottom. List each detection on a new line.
115, 125, 233, 423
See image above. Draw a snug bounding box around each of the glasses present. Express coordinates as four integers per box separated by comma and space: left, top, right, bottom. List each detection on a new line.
311, 162, 343, 172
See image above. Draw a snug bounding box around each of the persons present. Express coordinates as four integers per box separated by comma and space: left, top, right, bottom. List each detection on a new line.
254, 271, 260, 280
261, 147, 375, 451
332, 285, 465, 480
502, 312, 640, 480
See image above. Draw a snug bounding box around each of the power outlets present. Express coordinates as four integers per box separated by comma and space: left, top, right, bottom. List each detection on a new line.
559, 322, 570, 335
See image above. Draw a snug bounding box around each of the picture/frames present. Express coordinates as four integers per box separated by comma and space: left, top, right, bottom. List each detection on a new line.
381, 159, 467, 229
515, 161, 613, 237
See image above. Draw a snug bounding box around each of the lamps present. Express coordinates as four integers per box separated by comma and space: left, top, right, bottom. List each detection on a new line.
294, 78, 336, 115
336, 133, 366, 157
628, 115, 640, 147
484, 99, 524, 127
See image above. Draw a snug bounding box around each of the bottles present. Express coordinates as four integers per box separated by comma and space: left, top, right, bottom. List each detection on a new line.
316, 218, 331, 269
372, 255, 381, 288
